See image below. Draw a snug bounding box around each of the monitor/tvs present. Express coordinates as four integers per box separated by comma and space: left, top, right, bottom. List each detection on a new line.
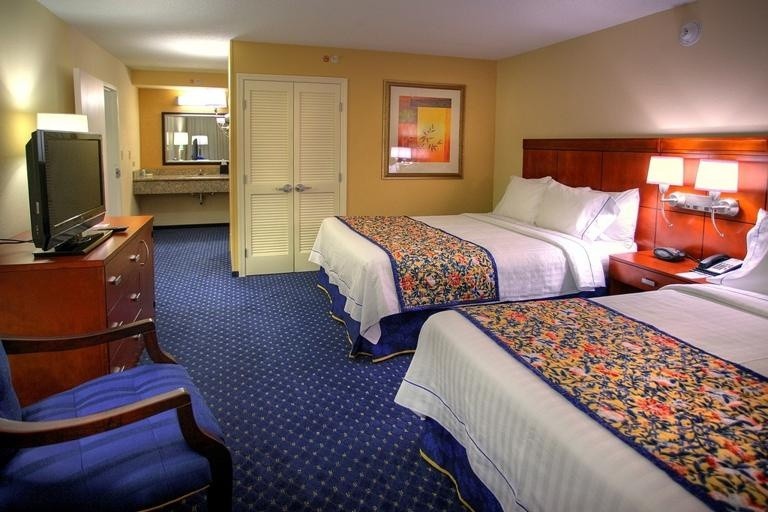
192, 138, 197, 159
26, 129, 113, 256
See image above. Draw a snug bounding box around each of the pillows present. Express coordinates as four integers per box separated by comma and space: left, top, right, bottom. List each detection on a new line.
492, 175, 640, 249
711, 210, 768, 297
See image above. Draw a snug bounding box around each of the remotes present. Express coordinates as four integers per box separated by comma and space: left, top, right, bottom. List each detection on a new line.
92, 225, 128, 232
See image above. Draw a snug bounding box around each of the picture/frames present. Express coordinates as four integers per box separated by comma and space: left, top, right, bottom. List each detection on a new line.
381, 79, 465, 181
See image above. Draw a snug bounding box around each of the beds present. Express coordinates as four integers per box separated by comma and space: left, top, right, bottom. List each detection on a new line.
308, 211, 637, 362
394, 278, 768, 512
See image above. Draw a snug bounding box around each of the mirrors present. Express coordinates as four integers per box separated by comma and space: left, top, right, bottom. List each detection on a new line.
161, 111, 229, 164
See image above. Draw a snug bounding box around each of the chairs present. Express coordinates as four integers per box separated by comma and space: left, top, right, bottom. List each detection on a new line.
0, 320, 234, 512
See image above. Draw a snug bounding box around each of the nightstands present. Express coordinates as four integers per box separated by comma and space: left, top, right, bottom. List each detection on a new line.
609, 252, 717, 296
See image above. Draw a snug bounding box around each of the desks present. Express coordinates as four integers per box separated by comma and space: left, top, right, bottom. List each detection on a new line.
0, 212, 153, 409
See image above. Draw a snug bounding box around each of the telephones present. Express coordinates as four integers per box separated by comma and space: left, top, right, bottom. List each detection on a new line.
696, 254, 744, 276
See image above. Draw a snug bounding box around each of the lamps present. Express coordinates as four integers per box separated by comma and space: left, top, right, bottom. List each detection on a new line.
174, 133, 189, 160
647, 155, 685, 228
694, 159, 739, 237
193, 136, 208, 159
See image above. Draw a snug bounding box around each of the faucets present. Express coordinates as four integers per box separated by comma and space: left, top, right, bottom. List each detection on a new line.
199, 168, 203, 175
